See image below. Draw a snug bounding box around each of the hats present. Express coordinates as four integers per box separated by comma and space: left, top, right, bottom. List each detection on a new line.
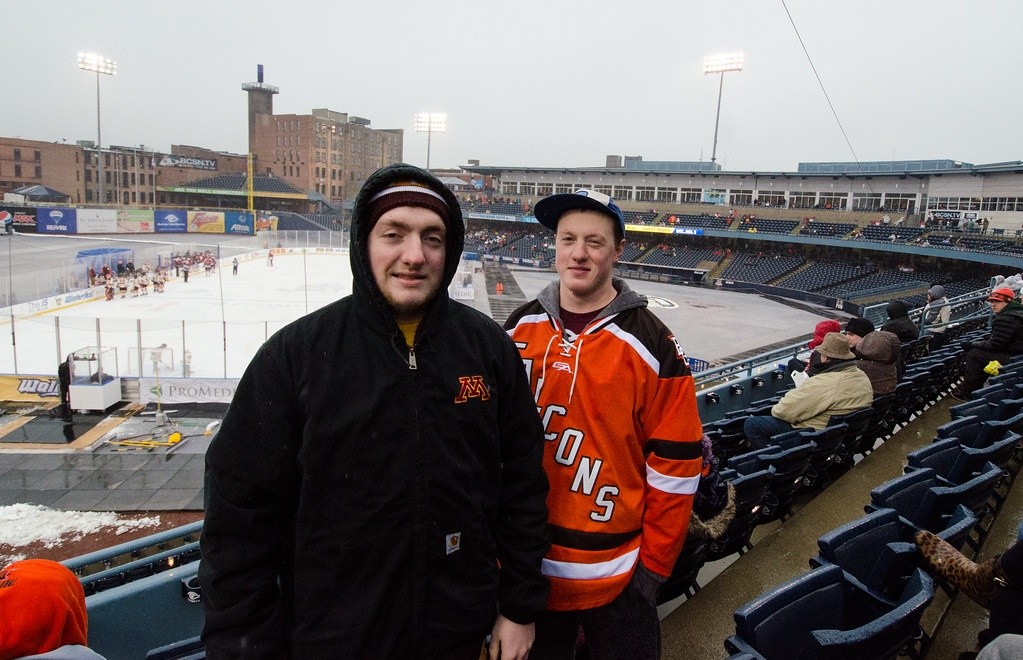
533, 188, 625, 238
845, 318, 875, 338
815, 331, 856, 359
808, 320, 841, 349
359, 179, 449, 236
986, 288, 1015, 302
0, 559, 89, 660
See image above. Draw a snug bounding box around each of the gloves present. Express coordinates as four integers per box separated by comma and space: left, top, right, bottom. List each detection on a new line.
960, 341, 972, 351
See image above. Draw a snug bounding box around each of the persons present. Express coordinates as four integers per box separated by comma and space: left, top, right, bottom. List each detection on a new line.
0, 559, 109, 660
678, 274, 1023, 659
499, 191, 705, 660
455, 191, 989, 285
88, 241, 283, 300
199, 161, 550, 660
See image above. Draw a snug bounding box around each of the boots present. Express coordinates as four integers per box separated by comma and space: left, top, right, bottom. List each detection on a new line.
915, 531, 1023, 610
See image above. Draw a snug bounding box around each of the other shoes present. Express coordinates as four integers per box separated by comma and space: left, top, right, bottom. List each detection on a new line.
950, 385, 973, 403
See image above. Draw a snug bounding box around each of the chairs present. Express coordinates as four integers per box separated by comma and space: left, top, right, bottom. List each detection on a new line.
84, 203, 1023, 660
